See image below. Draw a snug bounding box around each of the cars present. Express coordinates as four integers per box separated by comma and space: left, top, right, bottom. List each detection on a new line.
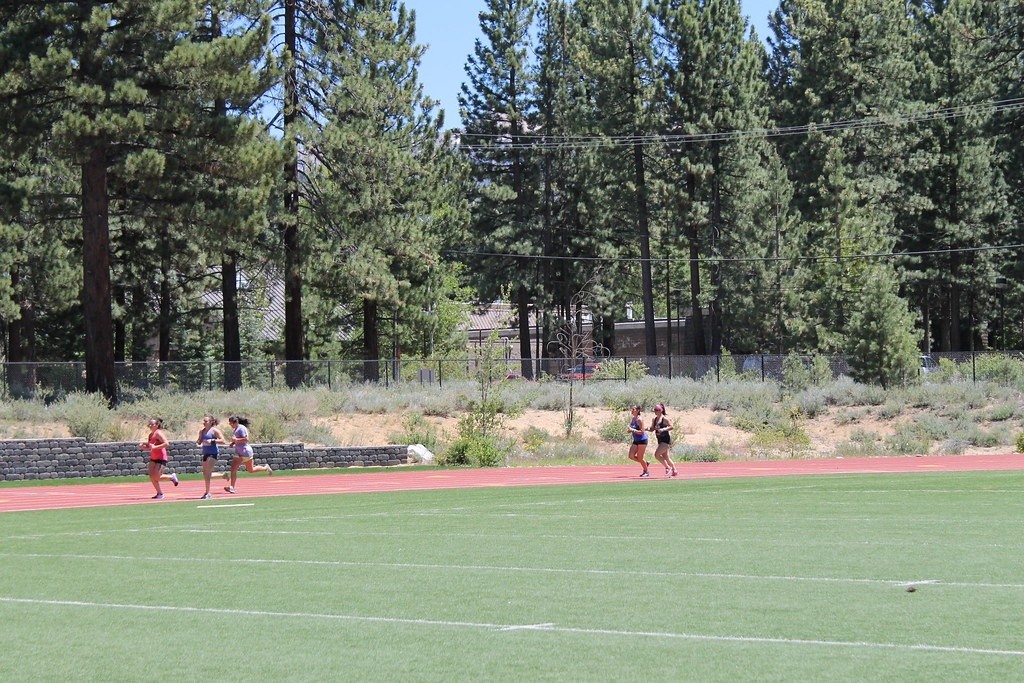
918, 356, 940, 373
491, 375, 529, 383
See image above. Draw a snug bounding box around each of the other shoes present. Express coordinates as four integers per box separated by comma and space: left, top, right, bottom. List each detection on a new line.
665, 467, 672, 476
669, 470, 679, 478
224, 471, 230, 481
201, 493, 208, 499
646, 461, 650, 467
640, 470, 649, 477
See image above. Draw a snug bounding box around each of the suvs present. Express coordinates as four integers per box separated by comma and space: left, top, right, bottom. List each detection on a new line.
559, 363, 601, 380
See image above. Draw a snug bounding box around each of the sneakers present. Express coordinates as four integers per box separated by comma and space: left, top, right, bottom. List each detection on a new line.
152, 494, 164, 499
265, 464, 272, 476
224, 486, 235, 493
170, 473, 178, 486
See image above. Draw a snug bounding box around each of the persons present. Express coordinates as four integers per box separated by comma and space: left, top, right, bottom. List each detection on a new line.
223, 417, 273, 493
645, 403, 679, 477
197, 416, 230, 500
139, 417, 179, 499
627, 405, 651, 477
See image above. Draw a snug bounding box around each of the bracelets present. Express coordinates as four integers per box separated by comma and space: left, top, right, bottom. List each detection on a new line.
631, 429, 636, 433
663, 428, 667, 432
211, 439, 215, 444
